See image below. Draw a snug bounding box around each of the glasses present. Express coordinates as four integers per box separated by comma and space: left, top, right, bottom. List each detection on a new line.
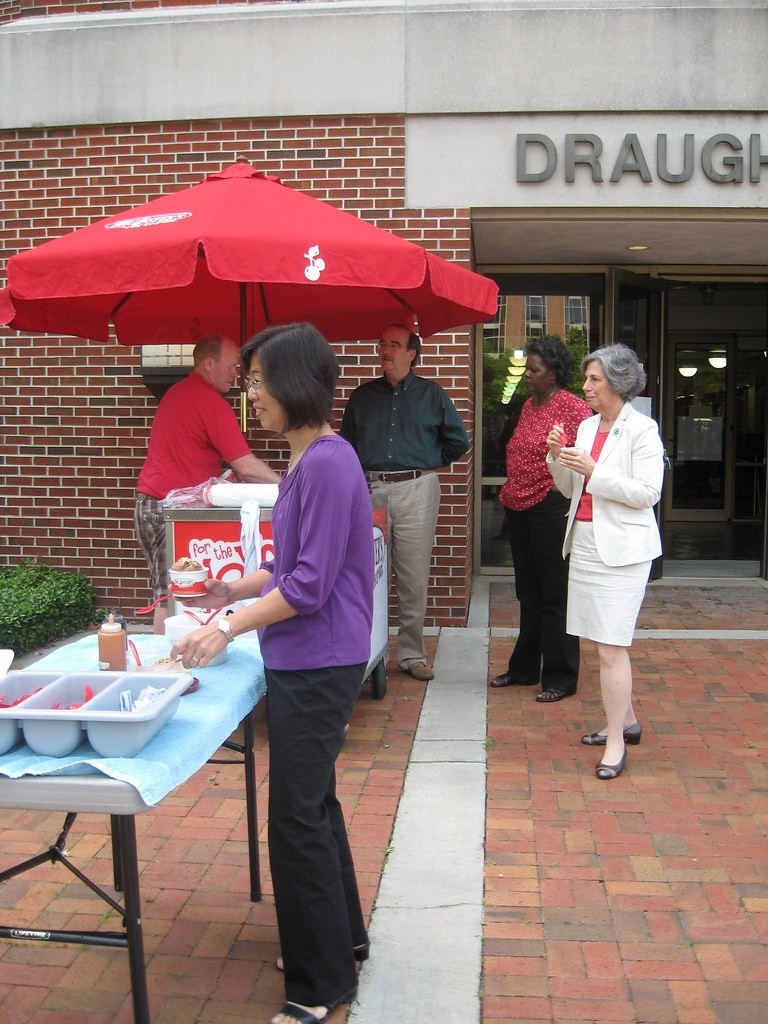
244, 377, 265, 394
377, 343, 407, 351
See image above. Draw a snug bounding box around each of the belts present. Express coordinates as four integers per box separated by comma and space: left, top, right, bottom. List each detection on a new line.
377, 471, 422, 484
137, 493, 158, 500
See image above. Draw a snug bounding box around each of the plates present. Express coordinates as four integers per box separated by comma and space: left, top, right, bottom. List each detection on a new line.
180, 677, 199, 695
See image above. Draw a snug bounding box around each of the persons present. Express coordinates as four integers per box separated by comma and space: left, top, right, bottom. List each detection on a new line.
170, 324, 374, 1024
490, 333, 592, 702
544, 344, 663, 779
133, 333, 281, 633
340, 323, 471, 680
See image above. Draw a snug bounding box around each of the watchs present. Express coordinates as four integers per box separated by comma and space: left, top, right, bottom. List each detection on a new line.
217, 618, 234, 643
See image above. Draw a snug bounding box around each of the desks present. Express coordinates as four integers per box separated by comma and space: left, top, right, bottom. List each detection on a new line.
0, 635, 268, 1024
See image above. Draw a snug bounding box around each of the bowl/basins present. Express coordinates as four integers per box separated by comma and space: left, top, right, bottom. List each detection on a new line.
163, 614, 227, 666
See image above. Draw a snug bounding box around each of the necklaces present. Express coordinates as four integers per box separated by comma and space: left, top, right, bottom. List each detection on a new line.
594, 433, 605, 453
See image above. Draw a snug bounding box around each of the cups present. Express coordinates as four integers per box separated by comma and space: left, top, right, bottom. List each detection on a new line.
220, 469, 240, 483
168, 567, 209, 597
560, 447, 585, 471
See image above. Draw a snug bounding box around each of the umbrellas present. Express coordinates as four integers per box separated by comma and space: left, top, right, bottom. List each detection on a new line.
0, 154, 499, 442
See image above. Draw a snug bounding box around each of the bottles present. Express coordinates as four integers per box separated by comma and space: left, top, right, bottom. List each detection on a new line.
98, 614, 127, 671
103, 609, 128, 651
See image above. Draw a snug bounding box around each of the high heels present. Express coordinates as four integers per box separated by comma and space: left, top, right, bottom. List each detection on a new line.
596, 740, 627, 779
271, 985, 357, 1024
276, 941, 369, 971
582, 721, 641, 745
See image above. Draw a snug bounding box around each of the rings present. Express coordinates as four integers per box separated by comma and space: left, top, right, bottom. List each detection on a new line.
192, 657, 199, 662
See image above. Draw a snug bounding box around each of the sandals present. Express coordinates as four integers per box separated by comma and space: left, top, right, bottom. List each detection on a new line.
536, 688, 573, 702
490, 674, 517, 687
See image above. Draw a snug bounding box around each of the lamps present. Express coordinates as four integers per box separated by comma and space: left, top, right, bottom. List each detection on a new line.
501, 348, 530, 405
709, 350, 727, 368
678, 350, 698, 377
702, 283, 715, 304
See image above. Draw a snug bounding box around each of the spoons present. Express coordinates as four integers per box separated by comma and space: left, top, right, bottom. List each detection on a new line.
134, 594, 172, 614
554, 418, 568, 444
129, 640, 146, 672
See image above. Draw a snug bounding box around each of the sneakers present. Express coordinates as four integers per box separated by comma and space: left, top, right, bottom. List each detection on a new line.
408, 661, 434, 681
